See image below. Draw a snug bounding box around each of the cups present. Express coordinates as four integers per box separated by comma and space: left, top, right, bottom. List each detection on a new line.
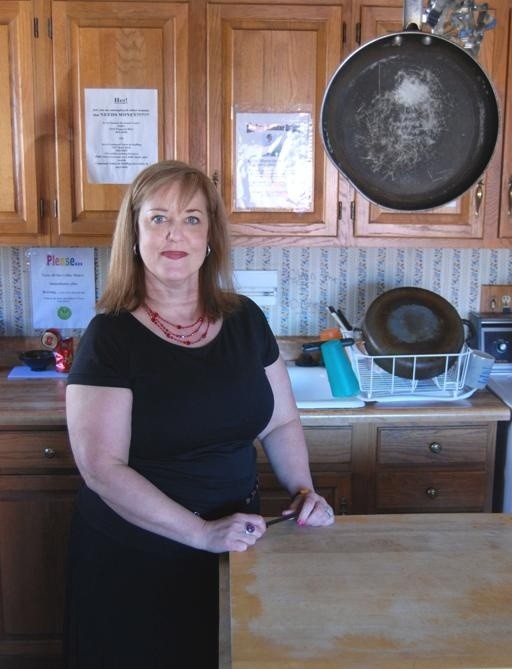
464, 350, 496, 391
320, 328, 361, 399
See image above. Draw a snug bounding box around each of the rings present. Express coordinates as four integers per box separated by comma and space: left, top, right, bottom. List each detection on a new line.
244, 521, 257, 536
323, 506, 335, 518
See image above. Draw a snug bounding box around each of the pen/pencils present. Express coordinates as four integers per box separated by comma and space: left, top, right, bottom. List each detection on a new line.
266, 513, 299, 525
325, 305, 352, 331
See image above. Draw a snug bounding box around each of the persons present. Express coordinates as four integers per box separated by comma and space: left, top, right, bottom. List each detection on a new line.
63, 158, 338, 667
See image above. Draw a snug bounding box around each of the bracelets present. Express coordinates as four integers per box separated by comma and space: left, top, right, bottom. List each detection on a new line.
291, 487, 314, 499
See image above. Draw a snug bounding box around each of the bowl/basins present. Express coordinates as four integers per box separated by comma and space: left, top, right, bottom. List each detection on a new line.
18, 349, 55, 372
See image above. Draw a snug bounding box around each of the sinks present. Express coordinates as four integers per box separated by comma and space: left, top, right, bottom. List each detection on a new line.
287, 364, 368, 409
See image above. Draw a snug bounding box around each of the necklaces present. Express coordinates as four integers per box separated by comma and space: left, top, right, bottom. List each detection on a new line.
142, 296, 213, 346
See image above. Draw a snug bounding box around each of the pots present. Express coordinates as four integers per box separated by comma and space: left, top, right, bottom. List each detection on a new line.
319, 1, 500, 214
301, 287, 464, 381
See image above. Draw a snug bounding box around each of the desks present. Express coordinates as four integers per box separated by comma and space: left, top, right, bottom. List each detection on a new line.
222, 514, 510, 669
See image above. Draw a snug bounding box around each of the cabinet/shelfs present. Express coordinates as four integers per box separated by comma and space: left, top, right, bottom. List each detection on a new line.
1, 0, 46, 244
48, 2, 347, 244
252, 425, 497, 515
349, 1, 511, 243
0, 429, 87, 669
